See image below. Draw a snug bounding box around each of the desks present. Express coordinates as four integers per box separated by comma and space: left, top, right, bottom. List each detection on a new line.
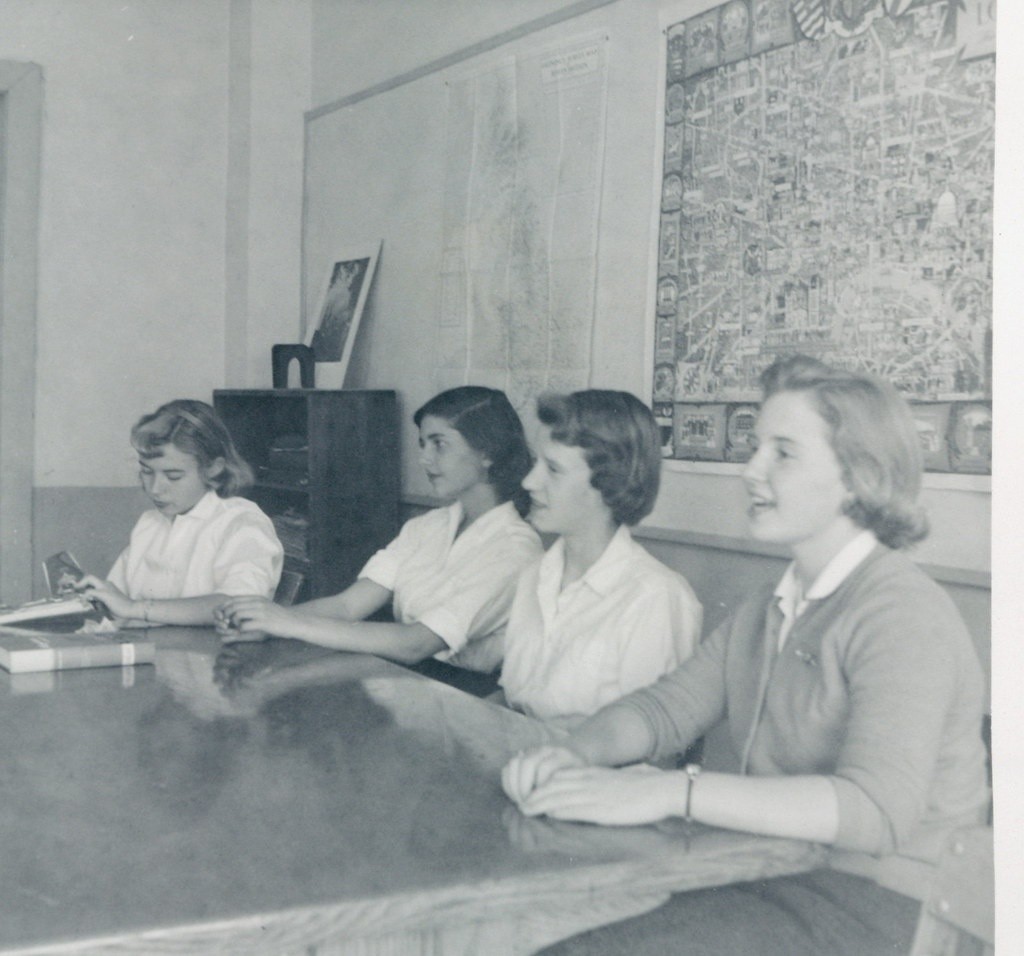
0, 618, 820, 956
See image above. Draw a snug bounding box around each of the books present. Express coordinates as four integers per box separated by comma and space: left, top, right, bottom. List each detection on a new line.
1, 589, 158, 675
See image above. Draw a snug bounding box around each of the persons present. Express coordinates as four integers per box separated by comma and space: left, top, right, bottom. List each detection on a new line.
500, 351, 994, 956
54, 397, 283, 630
481, 388, 702, 736
211, 385, 546, 699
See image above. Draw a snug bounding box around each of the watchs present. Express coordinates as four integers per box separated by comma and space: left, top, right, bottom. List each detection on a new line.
685, 764, 701, 826
143, 598, 155, 622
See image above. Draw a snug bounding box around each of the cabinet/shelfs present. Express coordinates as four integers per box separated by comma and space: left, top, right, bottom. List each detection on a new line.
215, 389, 400, 607
